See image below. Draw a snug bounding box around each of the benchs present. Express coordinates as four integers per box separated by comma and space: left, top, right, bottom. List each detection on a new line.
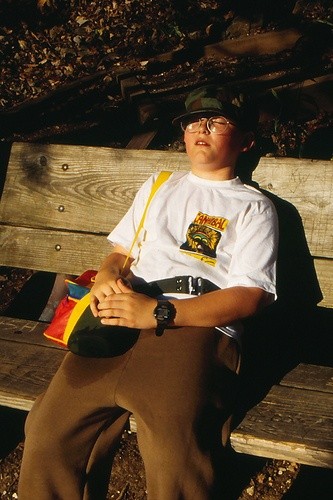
0, 142, 333, 469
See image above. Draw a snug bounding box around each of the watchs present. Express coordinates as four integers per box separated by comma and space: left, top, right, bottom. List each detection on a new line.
154, 300, 175, 336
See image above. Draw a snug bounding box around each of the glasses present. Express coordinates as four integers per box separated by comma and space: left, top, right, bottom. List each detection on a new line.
181, 115, 240, 134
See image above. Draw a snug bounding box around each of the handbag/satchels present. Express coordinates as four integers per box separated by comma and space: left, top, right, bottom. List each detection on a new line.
43, 270, 141, 358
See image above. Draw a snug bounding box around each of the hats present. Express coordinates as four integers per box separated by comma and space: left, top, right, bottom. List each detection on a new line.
172, 84, 256, 134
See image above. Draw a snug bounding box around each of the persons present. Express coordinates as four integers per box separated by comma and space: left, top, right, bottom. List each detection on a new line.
18, 83, 278, 500
